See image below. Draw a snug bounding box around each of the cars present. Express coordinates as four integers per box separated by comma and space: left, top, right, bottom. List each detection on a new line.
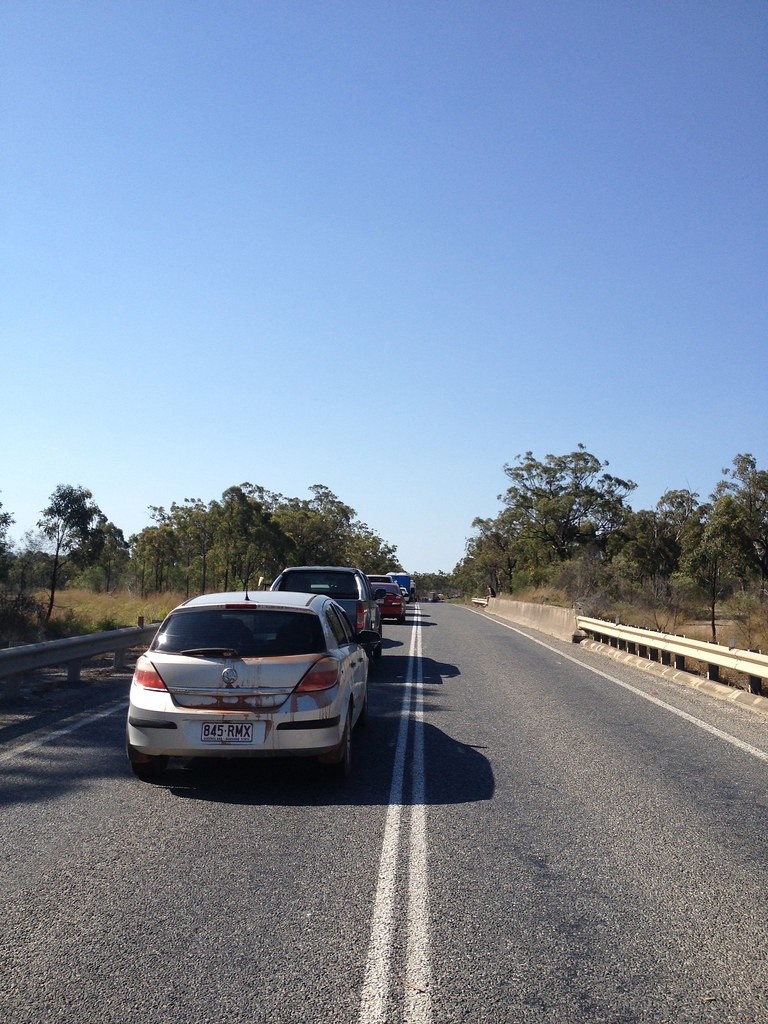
368, 583, 409, 621
125, 590, 382, 784
367, 572, 417, 604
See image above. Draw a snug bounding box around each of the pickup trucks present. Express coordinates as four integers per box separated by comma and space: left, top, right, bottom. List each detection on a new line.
268, 566, 382, 659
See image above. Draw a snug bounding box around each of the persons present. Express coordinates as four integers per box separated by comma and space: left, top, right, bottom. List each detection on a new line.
482, 584, 496, 607
